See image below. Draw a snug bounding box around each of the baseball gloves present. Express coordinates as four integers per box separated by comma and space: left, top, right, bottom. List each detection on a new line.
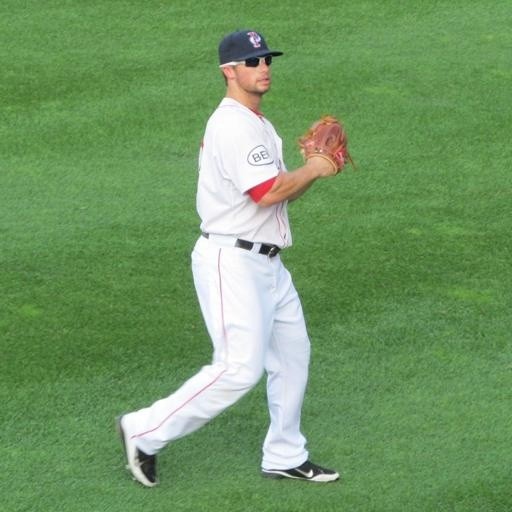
301, 116, 349, 176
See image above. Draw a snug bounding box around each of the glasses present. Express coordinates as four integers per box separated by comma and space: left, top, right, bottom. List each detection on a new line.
219, 56, 272, 67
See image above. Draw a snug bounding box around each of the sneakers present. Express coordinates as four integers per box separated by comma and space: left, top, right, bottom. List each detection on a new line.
119, 415, 157, 487
260, 460, 340, 482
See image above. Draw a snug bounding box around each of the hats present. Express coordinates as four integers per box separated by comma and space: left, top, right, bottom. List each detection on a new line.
218, 30, 283, 65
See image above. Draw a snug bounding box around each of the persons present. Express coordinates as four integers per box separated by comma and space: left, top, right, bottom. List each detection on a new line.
115, 27, 348, 490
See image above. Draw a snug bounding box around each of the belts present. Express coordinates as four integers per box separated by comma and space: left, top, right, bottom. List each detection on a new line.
200, 231, 281, 258
